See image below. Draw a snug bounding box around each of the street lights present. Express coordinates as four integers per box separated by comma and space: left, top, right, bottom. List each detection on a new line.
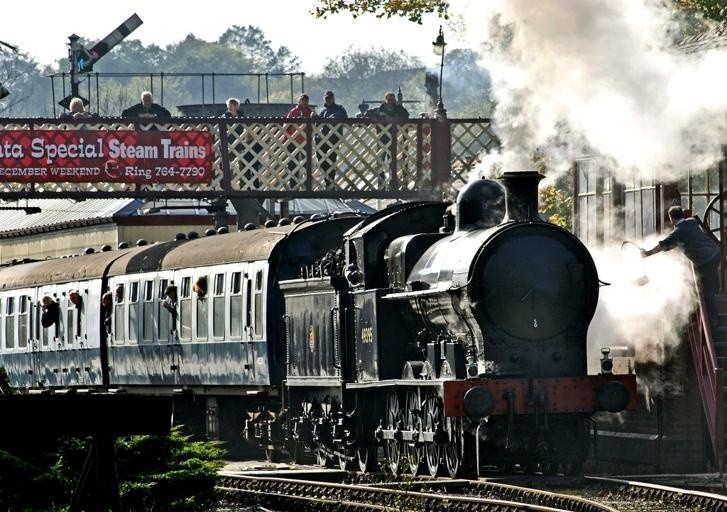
431, 23, 448, 120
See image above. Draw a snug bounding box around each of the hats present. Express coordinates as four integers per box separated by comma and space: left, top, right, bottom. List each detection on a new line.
300, 94, 309, 100
163, 285, 175, 295
323, 90, 333, 97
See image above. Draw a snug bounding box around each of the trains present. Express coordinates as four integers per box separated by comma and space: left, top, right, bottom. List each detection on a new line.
0, 170, 640, 479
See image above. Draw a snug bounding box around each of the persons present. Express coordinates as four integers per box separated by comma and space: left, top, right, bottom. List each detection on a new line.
69, 293, 82, 307
120, 91, 171, 132
278, 94, 317, 190
638, 205, 722, 330
216, 98, 249, 175
39, 295, 59, 330
60, 97, 99, 121
365, 92, 409, 191
161, 285, 177, 329
100, 292, 112, 309
310, 91, 348, 189
194, 275, 206, 300
115, 285, 124, 301
